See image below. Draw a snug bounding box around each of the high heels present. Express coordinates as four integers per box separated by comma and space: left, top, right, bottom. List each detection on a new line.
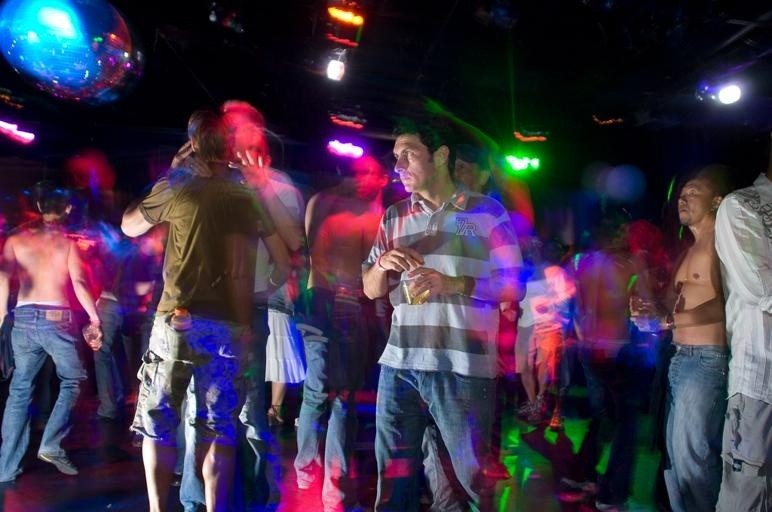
267, 406, 285, 427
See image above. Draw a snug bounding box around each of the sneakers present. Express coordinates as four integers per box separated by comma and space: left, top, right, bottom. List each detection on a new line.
550, 411, 563, 432
37, 452, 79, 475
516, 394, 549, 423
0, 464, 25, 484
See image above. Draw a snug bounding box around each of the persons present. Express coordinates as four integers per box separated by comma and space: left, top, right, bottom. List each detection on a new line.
1, 100, 772, 512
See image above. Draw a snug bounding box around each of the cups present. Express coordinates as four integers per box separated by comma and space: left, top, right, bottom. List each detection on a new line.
401, 270, 430, 305
82, 324, 99, 347
